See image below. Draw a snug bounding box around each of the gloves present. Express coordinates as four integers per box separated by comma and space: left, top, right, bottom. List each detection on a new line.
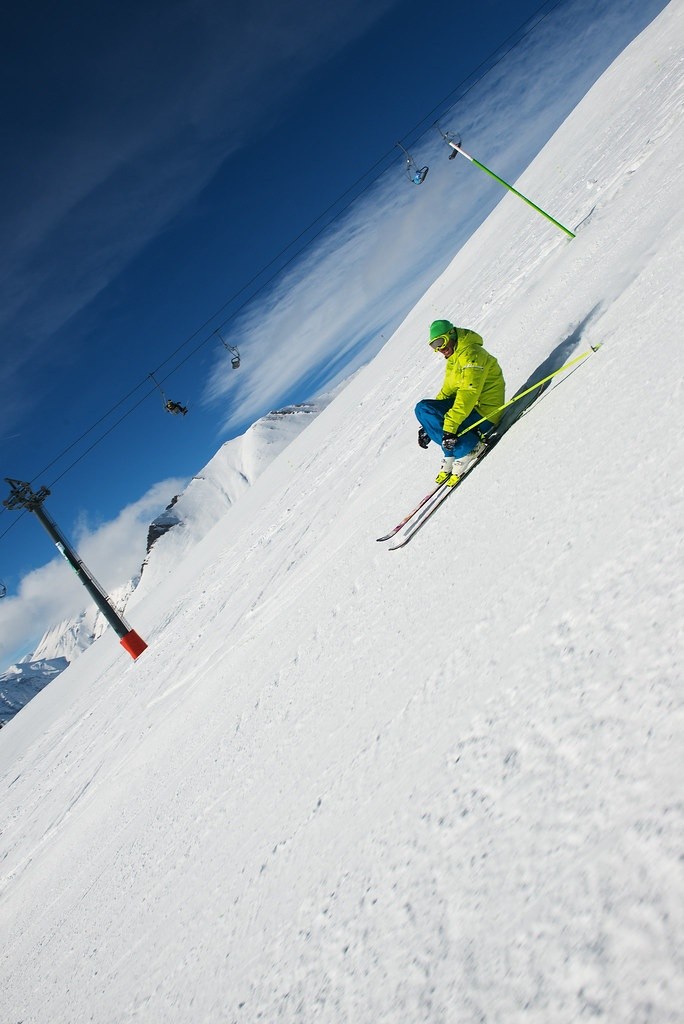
419, 428, 431, 449
442, 431, 458, 450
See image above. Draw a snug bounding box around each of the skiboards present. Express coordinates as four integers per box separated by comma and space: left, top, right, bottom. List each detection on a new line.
375, 380, 549, 552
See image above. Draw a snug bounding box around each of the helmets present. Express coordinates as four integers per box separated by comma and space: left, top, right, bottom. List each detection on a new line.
429, 320, 453, 340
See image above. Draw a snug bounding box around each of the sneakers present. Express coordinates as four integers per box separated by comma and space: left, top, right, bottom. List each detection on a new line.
452, 441, 486, 476
440, 454, 455, 473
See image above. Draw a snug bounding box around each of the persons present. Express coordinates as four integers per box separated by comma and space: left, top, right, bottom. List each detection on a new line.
168, 400, 189, 416
414, 319, 505, 487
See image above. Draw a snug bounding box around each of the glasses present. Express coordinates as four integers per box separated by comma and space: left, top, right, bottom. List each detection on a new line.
429, 334, 449, 352
